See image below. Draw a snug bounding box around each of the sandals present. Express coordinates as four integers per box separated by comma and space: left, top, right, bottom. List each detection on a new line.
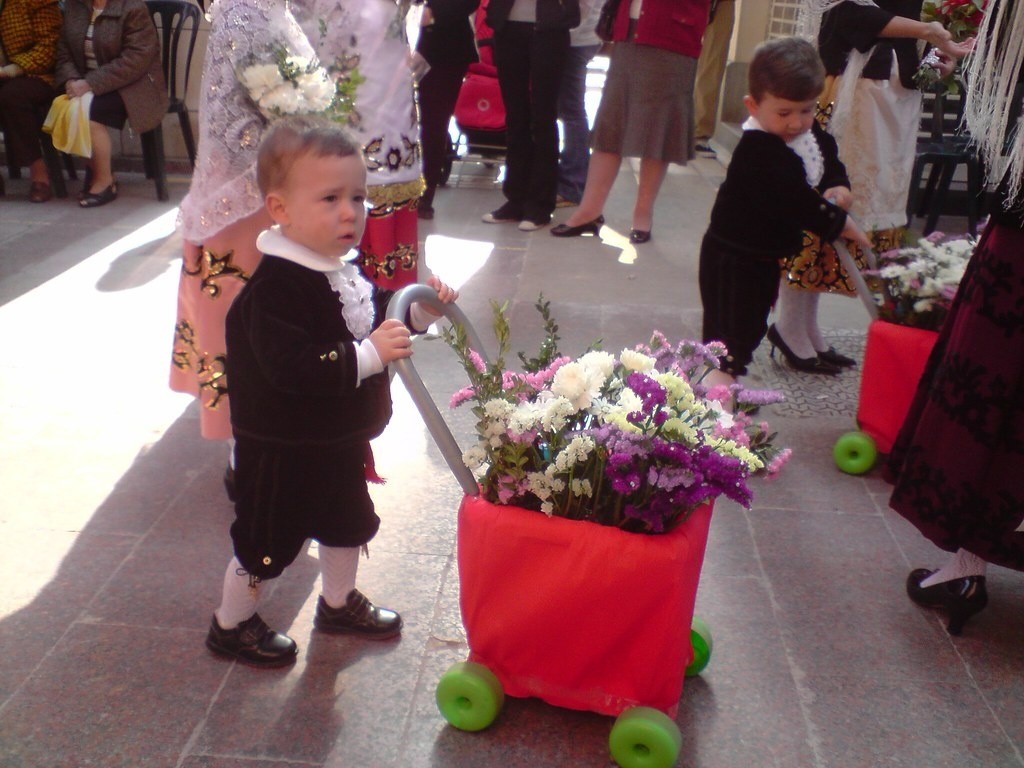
79, 181, 117, 208
82, 168, 94, 193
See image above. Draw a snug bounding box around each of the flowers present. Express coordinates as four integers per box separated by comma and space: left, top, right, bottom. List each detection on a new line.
862, 214, 992, 329
235, 54, 368, 134
911, 0, 992, 94
438, 292, 792, 535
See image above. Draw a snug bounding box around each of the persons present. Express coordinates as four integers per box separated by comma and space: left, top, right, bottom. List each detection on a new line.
56, 0, 168, 208
409, 0, 482, 220
482, 0, 581, 230
886, 0, 1024, 640
551, 0, 722, 243
170, 0, 422, 495
699, 34, 870, 417
207, 116, 460, 670
557, 0, 607, 209
766, 0, 975, 371
0, 0, 63, 204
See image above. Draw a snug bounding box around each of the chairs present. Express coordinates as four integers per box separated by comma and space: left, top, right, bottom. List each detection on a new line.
82, 0, 201, 202
905, 78, 984, 239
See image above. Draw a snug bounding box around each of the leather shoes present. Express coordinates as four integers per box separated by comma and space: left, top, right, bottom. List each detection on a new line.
314, 589, 402, 638
205, 610, 299, 666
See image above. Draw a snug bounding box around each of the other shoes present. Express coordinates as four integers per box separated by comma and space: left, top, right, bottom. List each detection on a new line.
417, 205, 434, 218
694, 144, 716, 158
518, 220, 545, 230
481, 212, 516, 222
553, 191, 579, 208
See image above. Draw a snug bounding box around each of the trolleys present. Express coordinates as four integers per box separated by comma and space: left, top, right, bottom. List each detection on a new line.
832, 236, 980, 476
386, 282, 714, 768
438, 37, 507, 187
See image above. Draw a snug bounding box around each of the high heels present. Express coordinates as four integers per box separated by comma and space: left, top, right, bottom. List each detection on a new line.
630, 227, 652, 243
767, 322, 841, 374
814, 345, 856, 367
906, 568, 987, 635
550, 215, 604, 237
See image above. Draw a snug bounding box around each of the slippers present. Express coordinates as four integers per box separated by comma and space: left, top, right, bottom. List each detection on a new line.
28, 179, 52, 202
